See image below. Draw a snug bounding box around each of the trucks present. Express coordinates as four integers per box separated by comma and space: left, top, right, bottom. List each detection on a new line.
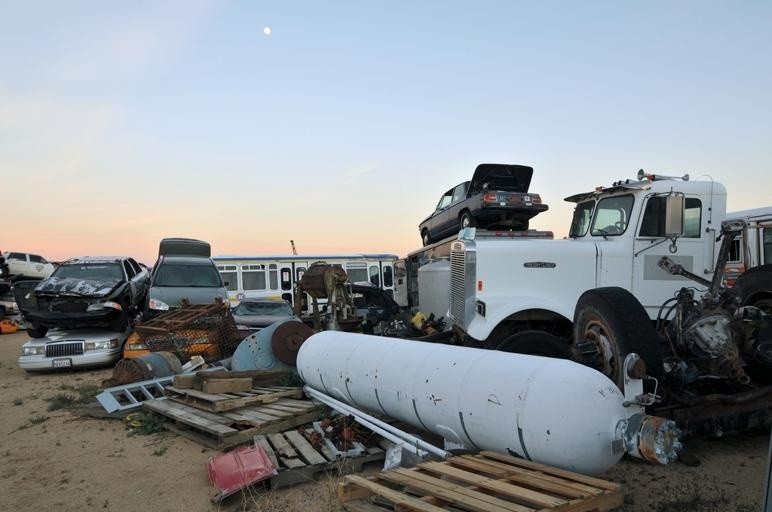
406, 168, 772, 450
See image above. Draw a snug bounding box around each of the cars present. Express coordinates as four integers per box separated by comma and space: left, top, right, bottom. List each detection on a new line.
418, 162, 550, 247
2, 237, 406, 374
